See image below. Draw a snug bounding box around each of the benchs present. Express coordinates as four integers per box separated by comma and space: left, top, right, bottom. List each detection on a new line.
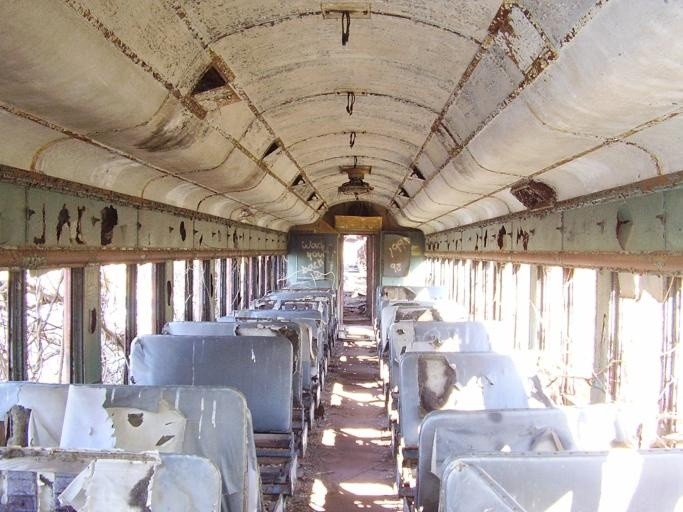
373, 285, 682, 510
0, 277, 336, 511
